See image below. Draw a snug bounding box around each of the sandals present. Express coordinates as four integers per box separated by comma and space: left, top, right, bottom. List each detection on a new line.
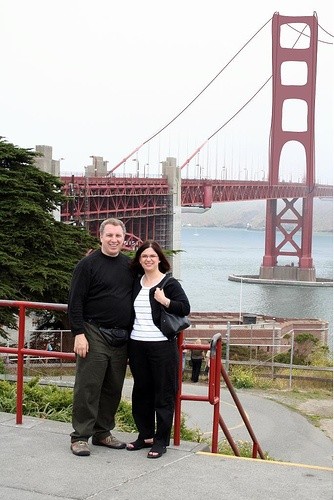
126, 438, 154, 451
147, 445, 167, 458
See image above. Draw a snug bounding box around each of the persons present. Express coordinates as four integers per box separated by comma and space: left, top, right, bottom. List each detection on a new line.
181, 338, 187, 382
190, 338, 205, 383
67, 217, 142, 456
85, 239, 191, 459
205, 349, 211, 383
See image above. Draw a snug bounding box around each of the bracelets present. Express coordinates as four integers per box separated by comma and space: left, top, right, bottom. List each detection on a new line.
165, 298, 169, 306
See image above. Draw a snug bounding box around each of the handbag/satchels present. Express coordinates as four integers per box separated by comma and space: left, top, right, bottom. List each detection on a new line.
98, 324, 129, 348
159, 277, 190, 337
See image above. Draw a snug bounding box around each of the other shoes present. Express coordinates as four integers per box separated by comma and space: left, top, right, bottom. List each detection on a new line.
92, 435, 126, 449
71, 441, 91, 456
